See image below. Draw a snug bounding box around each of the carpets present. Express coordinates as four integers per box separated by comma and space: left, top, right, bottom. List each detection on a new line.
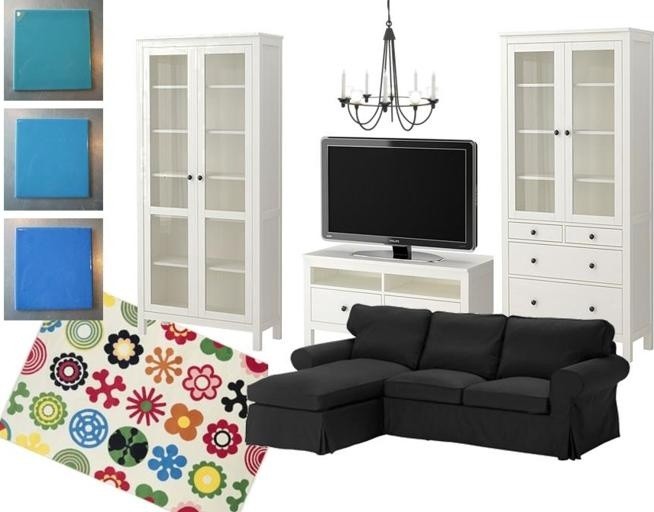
0, 291, 269, 512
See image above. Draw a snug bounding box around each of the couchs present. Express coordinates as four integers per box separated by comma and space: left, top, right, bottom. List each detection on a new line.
247, 303, 629, 462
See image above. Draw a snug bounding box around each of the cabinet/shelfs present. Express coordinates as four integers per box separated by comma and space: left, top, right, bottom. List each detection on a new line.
135, 31, 283, 351
498, 26, 654, 363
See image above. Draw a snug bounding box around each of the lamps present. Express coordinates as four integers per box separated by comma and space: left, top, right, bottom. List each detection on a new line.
338, 0, 439, 131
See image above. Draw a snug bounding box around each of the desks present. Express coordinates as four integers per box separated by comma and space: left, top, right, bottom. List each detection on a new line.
301, 243, 495, 348
